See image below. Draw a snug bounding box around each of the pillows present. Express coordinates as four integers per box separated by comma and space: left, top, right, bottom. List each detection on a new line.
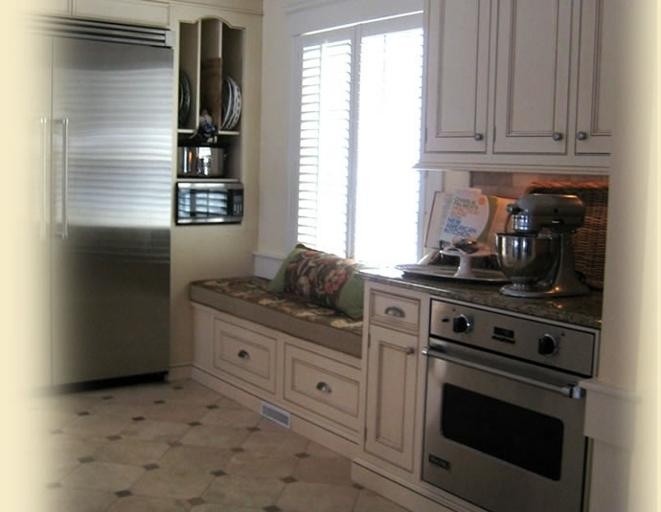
266, 241, 373, 323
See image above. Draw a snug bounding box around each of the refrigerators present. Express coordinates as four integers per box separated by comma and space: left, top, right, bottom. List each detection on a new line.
36, 35, 178, 389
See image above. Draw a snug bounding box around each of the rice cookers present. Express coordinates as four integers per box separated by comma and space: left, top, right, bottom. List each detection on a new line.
177, 136, 230, 178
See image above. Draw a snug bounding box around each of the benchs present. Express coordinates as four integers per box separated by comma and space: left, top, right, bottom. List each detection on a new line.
188, 276, 364, 456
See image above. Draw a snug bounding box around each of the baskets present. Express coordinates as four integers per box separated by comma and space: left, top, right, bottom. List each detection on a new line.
522, 180, 609, 288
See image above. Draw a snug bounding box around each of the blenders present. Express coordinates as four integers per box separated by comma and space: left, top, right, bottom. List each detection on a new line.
491, 189, 588, 299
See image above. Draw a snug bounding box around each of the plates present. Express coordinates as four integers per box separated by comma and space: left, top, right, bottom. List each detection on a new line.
179, 70, 192, 130
222, 74, 243, 133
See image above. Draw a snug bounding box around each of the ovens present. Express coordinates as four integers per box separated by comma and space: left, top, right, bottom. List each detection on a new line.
420, 298, 598, 512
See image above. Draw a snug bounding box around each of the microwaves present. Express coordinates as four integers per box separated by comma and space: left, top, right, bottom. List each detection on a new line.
174, 181, 244, 227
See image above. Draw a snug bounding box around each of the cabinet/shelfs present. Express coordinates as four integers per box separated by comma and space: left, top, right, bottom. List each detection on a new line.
176, 13, 246, 185
412, 0, 492, 172
362, 278, 429, 488
489, 1, 615, 175
